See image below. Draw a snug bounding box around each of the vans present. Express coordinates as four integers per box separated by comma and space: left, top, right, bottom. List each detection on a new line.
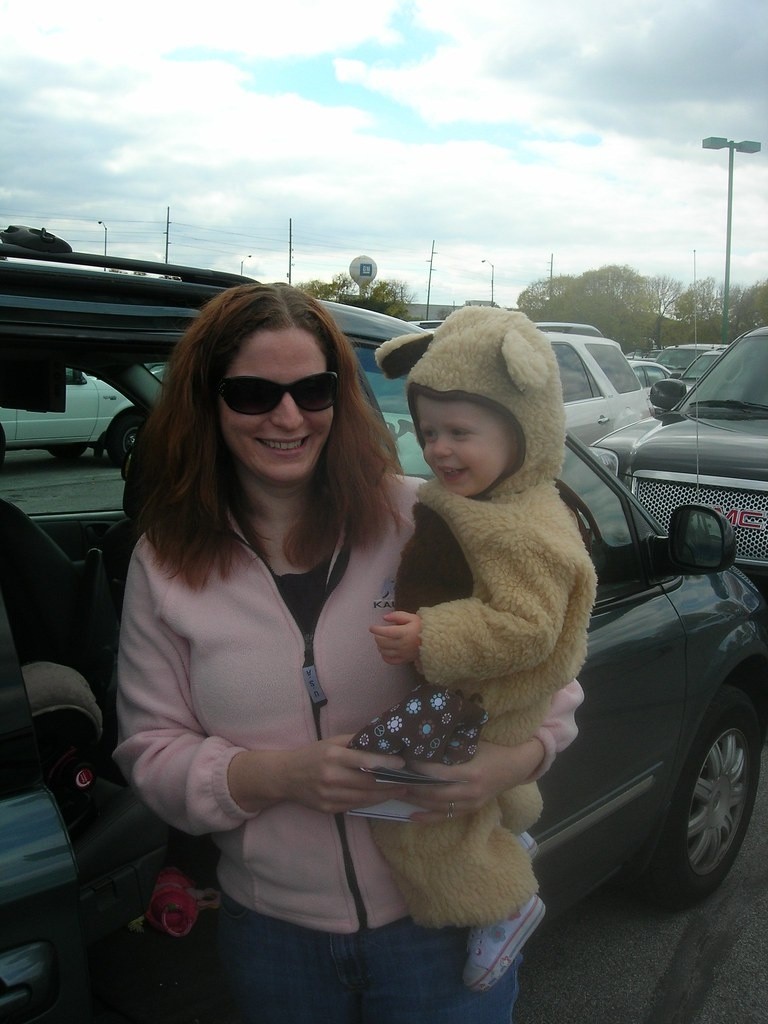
533, 322, 617, 395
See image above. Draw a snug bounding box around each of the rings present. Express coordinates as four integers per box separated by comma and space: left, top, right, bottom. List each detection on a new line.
444, 811, 454, 823
447, 801, 455, 813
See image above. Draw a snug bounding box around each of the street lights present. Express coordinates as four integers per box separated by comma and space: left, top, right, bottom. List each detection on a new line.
240, 254, 252, 276
702, 135, 761, 349
481, 259, 496, 307
98, 220, 109, 271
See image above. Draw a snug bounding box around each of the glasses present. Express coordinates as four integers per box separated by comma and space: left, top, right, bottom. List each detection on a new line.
217, 371, 339, 416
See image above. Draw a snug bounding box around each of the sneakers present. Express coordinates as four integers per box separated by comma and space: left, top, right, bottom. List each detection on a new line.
462, 894, 546, 992
517, 831, 539, 859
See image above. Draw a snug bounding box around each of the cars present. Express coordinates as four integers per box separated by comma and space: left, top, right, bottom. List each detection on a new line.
623, 342, 667, 364
626, 360, 677, 415
0, 367, 153, 475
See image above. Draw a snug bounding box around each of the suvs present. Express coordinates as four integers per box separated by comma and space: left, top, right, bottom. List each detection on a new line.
0, 224, 768, 1024
360, 318, 655, 478
654, 347, 747, 412
655, 344, 734, 378
588, 324, 768, 572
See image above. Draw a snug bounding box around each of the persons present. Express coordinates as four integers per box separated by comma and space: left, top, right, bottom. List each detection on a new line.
368, 304, 600, 989
110, 282, 587, 1024
